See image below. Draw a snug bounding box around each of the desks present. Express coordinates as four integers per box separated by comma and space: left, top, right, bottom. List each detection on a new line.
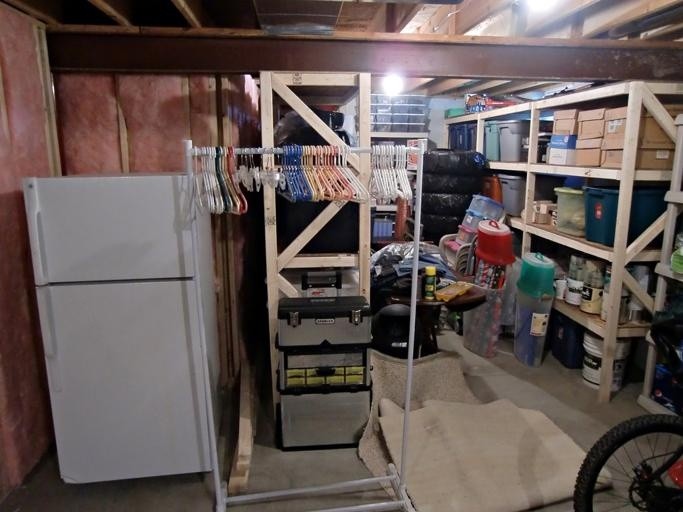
390, 282, 486, 334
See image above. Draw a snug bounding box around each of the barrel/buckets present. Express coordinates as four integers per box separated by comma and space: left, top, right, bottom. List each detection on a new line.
582, 331, 633, 392
551, 314, 584, 369
580, 280, 603, 315
601, 291, 631, 324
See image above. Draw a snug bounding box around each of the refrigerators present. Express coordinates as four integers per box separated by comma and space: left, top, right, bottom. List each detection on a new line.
20, 170, 224, 487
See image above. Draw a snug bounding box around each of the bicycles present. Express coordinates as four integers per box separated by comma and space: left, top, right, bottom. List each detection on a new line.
572, 414, 682, 512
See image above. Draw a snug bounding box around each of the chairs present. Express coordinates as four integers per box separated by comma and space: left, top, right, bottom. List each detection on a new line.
439, 213, 505, 275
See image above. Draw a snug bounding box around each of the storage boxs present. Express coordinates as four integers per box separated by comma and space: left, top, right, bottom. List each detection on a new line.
371, 93, 429, 132
545, 308, 586, 369
482, 174, 683, 248
455, 194, 504, 245
447, 104, 683, 170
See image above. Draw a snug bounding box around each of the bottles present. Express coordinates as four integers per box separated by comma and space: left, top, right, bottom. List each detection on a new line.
424, 265, 436, 301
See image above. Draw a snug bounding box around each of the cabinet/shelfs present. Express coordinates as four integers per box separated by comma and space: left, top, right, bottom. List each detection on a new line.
445, 79, 683, 403
638, 114, 683, 416
261, 70, 371, 419
372, 132, 428, 237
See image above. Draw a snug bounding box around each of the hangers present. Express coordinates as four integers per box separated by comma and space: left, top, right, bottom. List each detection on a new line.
189, 145, 413, 214
